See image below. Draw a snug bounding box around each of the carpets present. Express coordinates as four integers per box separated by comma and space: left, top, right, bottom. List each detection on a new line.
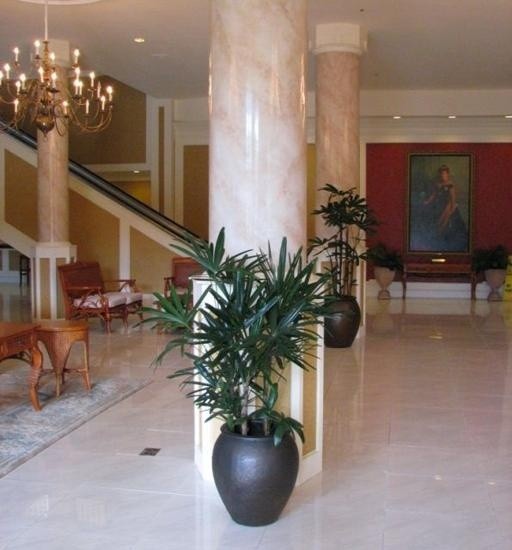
0, 363, 155, 476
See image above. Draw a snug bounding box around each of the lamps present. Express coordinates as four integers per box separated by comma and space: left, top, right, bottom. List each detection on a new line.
0, 1, 115, 144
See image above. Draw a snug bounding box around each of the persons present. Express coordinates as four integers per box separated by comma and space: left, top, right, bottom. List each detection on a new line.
423, 164, 468, 252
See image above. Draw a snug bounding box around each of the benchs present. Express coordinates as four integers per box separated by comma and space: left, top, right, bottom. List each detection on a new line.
56, 261, 144, 334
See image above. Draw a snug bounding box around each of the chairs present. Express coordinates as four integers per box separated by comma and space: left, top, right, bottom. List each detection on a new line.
154, 256, 206, 334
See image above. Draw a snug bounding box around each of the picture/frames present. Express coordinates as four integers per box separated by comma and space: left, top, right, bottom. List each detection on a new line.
401, 151, 476, 262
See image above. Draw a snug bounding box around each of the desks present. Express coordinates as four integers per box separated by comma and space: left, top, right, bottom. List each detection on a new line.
1, 319, 91, 411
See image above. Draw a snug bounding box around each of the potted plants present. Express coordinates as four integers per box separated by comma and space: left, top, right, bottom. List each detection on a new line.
470, 243, 509, 302
130, 226, 342, 526
307, 183, 405, 349
